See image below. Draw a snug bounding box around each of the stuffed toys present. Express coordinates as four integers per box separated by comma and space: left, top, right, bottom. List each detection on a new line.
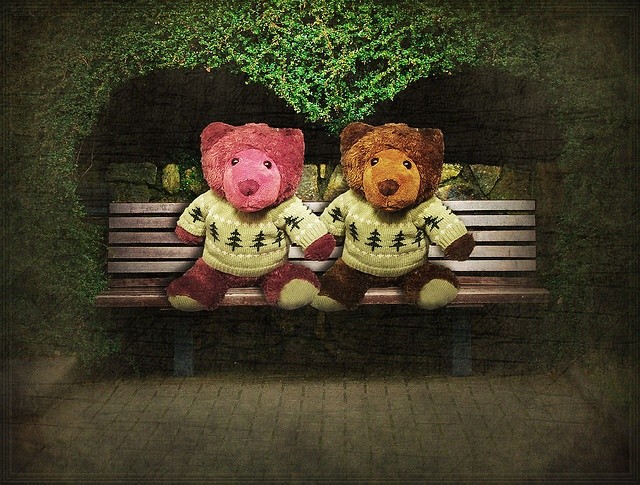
165, 122, 336, 313
321, 121, 474, 311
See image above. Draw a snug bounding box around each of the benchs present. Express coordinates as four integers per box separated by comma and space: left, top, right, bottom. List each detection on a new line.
95, 200, 551, 379
